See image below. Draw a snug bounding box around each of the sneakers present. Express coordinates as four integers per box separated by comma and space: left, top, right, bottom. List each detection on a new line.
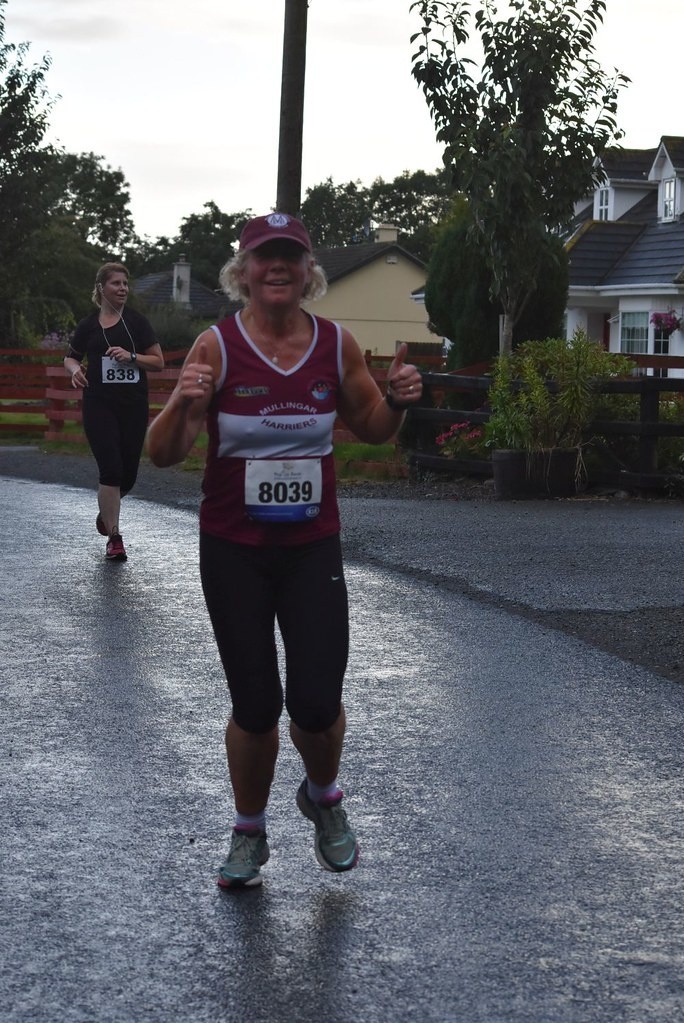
218, 825, 270, 891
296, 779, 359, 873
96, 513, 109, 536
106, 526, 127, 561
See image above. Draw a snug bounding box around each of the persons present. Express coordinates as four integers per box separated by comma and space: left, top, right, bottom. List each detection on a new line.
63, 262, 164, 560
146, 213, 423, 891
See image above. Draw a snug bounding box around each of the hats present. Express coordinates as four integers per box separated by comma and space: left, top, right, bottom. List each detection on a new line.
239, 213, 312, 258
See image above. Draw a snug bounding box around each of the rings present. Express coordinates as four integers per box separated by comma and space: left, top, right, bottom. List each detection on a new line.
199, 373, 203, 379
409, 385, 414, 394
197, 378, 202, 385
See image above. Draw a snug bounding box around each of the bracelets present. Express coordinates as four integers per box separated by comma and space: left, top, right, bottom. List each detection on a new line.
385, 385, 411, 410
129, 352, 137, 363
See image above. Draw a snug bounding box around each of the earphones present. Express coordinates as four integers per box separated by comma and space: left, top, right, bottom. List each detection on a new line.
99, 287, 102, 293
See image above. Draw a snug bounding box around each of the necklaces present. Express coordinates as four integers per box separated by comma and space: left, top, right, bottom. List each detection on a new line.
250, 310, 298, 364
